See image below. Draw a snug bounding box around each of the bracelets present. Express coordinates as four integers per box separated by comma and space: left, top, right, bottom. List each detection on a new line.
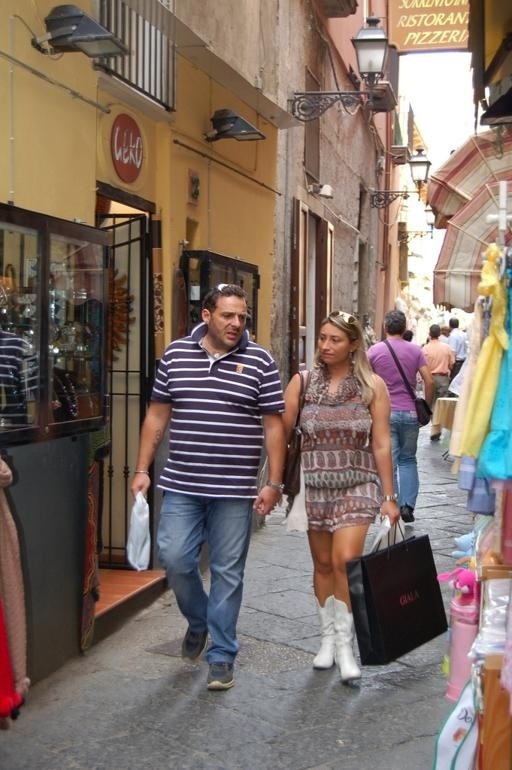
268, 479, 286, 494
135, 471, 151, 475
384, 493, 400, 502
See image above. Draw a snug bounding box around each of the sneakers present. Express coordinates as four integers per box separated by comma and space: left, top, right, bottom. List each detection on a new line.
182, 628, 208, 662
401, 506, 415, 522
207, 662, 234, 689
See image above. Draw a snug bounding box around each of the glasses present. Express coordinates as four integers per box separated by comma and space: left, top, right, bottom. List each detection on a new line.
329, 311, 359, 327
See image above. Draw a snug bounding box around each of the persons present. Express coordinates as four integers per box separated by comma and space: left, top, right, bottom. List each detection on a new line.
420, 319, 469, 442
132, 285, 285, 689
254, 309, 400, 683
367, 311, 436, 521
403, 330, 413, 341
0, 328, 40, 429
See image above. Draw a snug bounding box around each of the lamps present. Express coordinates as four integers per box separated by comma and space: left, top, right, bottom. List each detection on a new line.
368, 148, 431, 206
206, 106, 266, 140
285, 13, 391, 123
310, 183, 334, 198
29, 3, 134, 58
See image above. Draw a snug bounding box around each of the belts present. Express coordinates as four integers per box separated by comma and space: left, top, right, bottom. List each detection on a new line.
432, 373, 447, 375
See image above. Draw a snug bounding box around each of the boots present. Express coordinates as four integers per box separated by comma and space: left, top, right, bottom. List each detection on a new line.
334, 595, 361, 681
313, 595, 335, 669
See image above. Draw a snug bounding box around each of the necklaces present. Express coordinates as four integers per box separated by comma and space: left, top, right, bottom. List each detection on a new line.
53, 371, 80, 418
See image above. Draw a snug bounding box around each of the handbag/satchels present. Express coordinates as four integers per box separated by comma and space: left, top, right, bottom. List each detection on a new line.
284, 427, 302, 495
415, 398, 433, 425
344, 535, 448, 664
